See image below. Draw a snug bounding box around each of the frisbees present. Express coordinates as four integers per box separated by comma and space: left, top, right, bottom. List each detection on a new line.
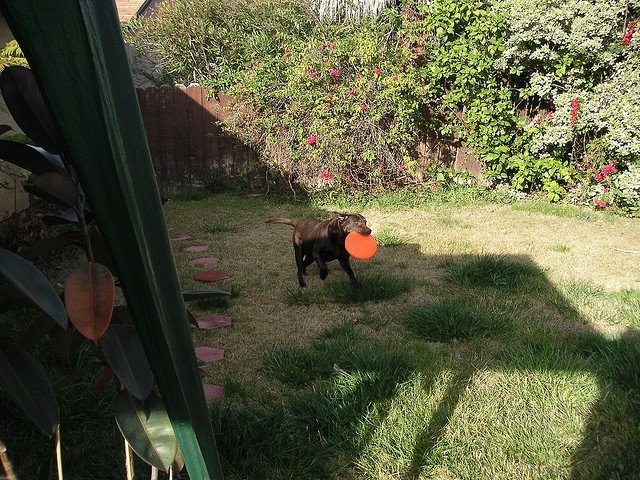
344, 232, 379, 260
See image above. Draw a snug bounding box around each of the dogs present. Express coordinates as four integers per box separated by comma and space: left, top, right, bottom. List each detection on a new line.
264, 213, 372, 288
